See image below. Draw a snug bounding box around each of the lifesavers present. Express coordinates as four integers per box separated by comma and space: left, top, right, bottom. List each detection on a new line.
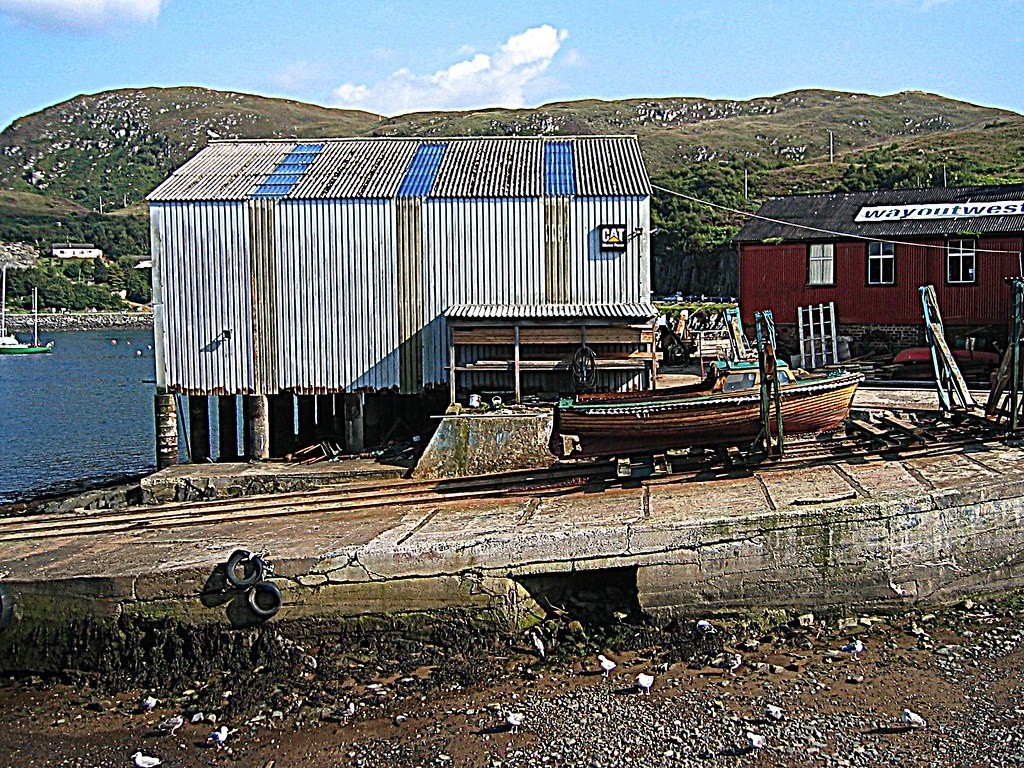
226, 552, 264, 588
249, 583, 283, 616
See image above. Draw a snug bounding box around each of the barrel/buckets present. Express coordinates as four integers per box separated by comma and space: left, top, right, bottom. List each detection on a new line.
469, 395, 481, 407
492, 396, 501, 406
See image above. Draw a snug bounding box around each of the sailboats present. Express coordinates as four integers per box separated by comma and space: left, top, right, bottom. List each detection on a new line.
0, 262, 54, 353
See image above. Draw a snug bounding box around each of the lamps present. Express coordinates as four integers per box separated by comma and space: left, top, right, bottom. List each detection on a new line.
633, 226, 645, 238
219, 328, 234, 343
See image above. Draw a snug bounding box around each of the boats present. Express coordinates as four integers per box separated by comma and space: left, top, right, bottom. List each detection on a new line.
893, 347, 1000, 368
548, 371, 868, 459
